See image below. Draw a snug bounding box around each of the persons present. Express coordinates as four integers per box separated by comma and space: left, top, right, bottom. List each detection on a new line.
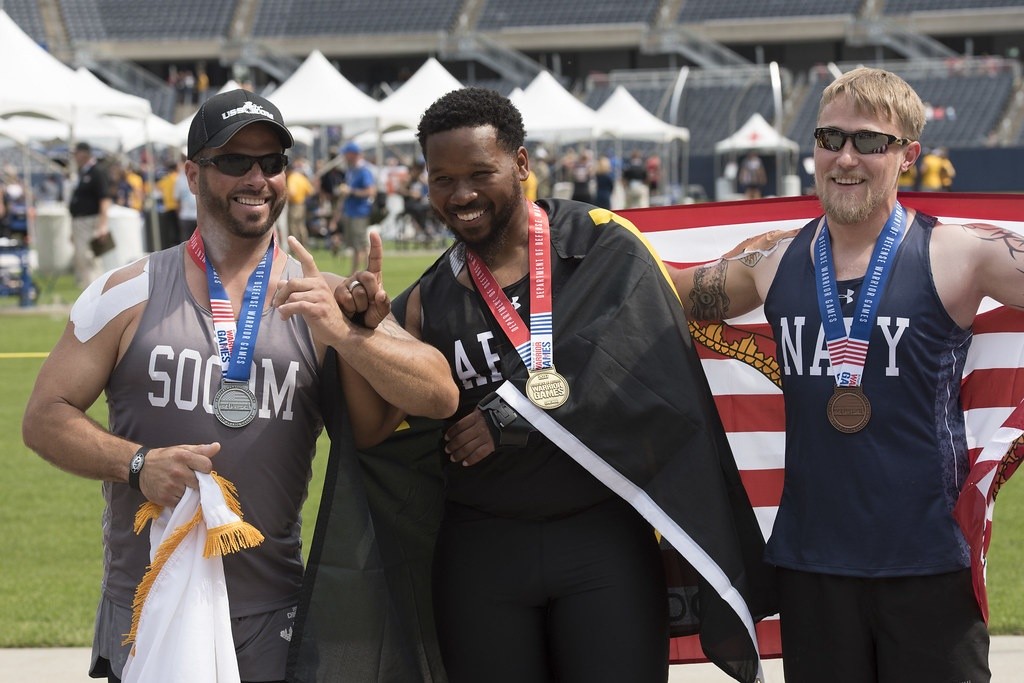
909, 144, 961, 199
330, 144, 374, 274
68, 143, 120, 291
22, 81, 461, 682
333, 85, 673, 680
658, 64, 1024, 683
122, 156, 199, 249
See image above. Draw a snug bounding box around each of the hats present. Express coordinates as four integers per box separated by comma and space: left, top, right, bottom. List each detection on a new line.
77, 143, 89, 149
338, 142, 362, 154
187, 89, 294, 159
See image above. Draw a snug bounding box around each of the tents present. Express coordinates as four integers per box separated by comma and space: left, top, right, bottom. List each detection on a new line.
0, 8, 171, 279
174, 53, 474, 155
704, 115, 803, 199
507, 77, 592, 204
584, 81, 690, 206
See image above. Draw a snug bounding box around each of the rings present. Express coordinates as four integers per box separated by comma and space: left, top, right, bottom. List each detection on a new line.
348, 280, 361, 292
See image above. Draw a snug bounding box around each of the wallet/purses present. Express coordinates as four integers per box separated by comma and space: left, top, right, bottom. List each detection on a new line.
87, 234, 113, 257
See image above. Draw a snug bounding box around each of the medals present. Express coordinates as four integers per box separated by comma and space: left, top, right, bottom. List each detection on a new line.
525, 368, 570, 411
213, 380, 261, 431
826, 386, 873, 435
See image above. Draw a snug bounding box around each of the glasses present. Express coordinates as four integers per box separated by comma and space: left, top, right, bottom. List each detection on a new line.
194, 153, 290, 177
813, 127, 912, 154
